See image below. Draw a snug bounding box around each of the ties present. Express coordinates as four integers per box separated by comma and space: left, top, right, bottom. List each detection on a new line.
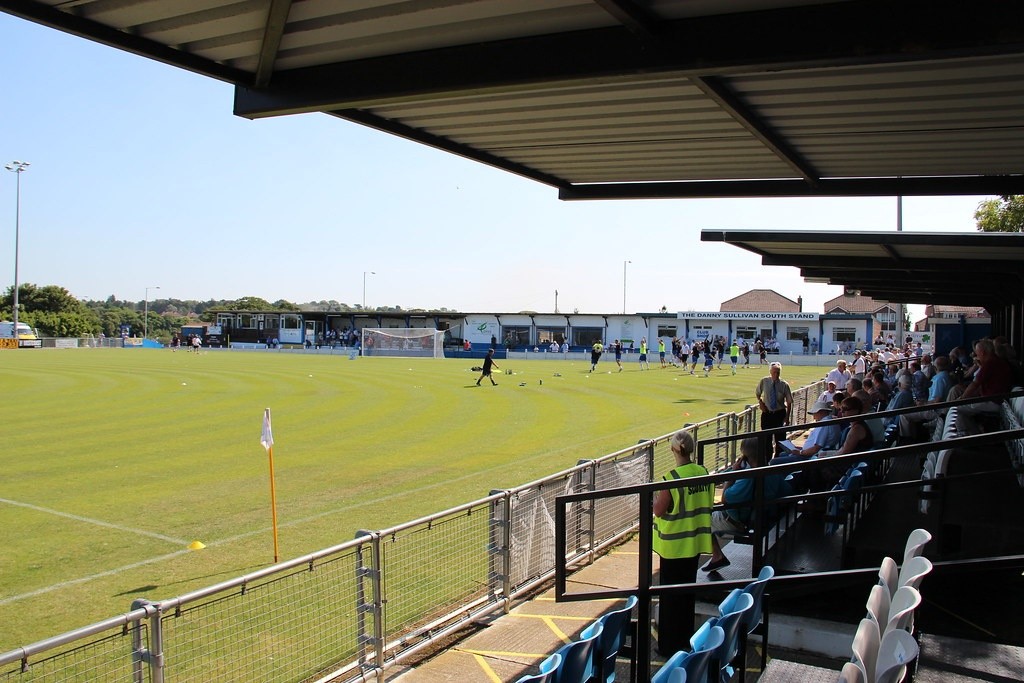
770, 383, 776, 409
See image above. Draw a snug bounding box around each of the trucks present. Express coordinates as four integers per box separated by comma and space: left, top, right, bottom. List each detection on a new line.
0, 321, 37, 348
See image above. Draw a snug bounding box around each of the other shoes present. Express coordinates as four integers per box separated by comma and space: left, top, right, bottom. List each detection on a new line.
654, 646, 670, 656
592, 366, 595, 371
590, 370, 591, 373
476, 383, 481, 386
493, 383, 498, 385
618, 365, 750, 377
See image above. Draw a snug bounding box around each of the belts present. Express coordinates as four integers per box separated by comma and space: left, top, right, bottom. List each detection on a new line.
766, 410, 784, 414
722, 509, 740, 528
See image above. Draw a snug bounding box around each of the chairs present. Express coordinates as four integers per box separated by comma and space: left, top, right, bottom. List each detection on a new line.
518, 385, 1024, 683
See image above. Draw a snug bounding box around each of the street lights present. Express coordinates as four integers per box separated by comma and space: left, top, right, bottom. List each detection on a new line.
5, 161, 31, 339
145, 286, 161, 339
363, 271, 376, 308
624, 261, 633, 314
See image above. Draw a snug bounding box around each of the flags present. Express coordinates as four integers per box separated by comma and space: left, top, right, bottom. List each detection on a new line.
261, 411, 275, 451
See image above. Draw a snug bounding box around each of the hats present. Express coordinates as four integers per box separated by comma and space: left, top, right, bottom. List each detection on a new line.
853, 350, 861, 355
828, 381, 836, 388
672, 431, 693, 453
806, 401, 832, 415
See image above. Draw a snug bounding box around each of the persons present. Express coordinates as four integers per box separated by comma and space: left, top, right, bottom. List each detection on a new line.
476, 348, 499, 386
615, 339, 622, 373
702, 334, 1024, 571
187, 333, 202, 354
504, 335, 512, 351
99, 332, 104, 346
173, 334, 179, 351
657, 337, 666, 368
671, 334, 780, 378
550, 341, 559, 353
364, 331, 429, 350
266, 336, 278, 348
802, 335, 818, 355
315, 326, 362, 349
305, 340, 311, 348
639, 336, 650, 371
560, 341, 568, 352
490, 334, 497, 354
590, 340, 603, 373
464, 339, 471, 350
653, 431, 713, 660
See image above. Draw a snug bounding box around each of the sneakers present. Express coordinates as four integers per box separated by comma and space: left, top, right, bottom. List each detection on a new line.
701, 556, 730, 572
